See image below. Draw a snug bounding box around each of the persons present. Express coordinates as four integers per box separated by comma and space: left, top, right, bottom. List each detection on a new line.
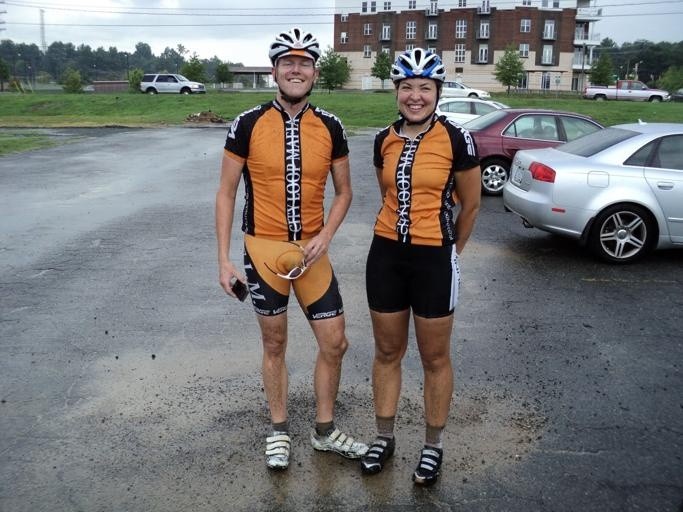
360, 47, 482, 486
215, 27, 370, 472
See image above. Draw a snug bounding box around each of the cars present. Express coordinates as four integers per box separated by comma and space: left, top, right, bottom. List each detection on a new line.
456, 108, 606, 195
433, 96, 559, 139
498, 118, 682, 262
673, 87, 682, 101
438, 80, 492, 100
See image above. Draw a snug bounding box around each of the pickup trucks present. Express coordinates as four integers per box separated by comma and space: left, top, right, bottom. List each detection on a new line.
582, 79, 671, 106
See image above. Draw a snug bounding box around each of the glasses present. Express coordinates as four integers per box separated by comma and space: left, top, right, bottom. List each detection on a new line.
264, 240, 311, 281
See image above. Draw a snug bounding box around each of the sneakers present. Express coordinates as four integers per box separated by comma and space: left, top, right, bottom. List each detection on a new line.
359, 434, 396, 477
263, 428, 292, 471
411, 443, 444, 487
308, 427, 370, 461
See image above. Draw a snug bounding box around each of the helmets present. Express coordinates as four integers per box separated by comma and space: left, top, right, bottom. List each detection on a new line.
388, 47, 447, 84
268, 26, 322, 67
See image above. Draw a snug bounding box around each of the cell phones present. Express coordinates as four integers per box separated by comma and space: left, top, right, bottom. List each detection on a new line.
230, 279, 249, 302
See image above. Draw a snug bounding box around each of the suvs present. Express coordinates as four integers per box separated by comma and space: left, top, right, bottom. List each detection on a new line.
138, 73, 206, 96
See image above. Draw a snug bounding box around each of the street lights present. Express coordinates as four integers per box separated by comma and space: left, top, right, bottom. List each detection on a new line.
616, 64, 623, 79
124, 51, 129, 80
634, 60, 643, 78
12, 53, 21, 78
